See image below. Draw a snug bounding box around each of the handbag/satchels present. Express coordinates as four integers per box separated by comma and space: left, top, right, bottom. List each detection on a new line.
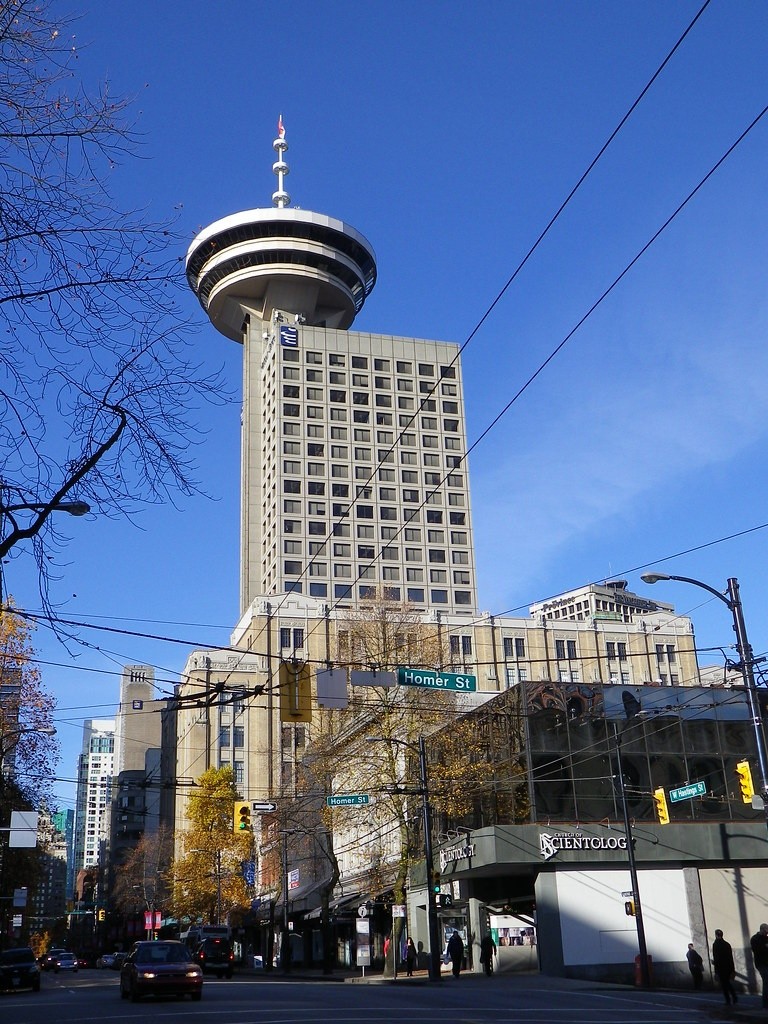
444, 956, 450, 965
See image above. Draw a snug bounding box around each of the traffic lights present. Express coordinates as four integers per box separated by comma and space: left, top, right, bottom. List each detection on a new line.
234, 802, 250, 834
652, 788, 670, 825
432, 871, 441, 893
439, 894, 452, 908
625, 901, 635, 916
734, 761, 755, 804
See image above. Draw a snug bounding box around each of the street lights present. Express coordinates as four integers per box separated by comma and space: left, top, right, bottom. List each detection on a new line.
365, 736, 441, 977
640, 573, 768, 795
190, 849, 221, 926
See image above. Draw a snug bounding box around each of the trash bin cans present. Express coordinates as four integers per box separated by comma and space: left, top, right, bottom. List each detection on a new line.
634, 954, 656, 990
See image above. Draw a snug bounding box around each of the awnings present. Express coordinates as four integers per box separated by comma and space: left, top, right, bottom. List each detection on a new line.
304, 885, 395, 920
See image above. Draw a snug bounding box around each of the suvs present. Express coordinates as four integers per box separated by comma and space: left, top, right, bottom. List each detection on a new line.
43, 949, 66, 969
113, 952, 127, 962
195, 937, 234, 979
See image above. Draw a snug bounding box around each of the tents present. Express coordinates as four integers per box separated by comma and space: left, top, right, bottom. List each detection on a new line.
256, 878, 330, 920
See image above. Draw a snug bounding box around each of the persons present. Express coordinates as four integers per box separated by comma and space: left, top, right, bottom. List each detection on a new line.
499, 928, 532, 946
750, 924, 768, 1007
475, 931, 496, 977
447, 930, 464, 979
403, 937, 418, 976
384, 936, 390, 955
713, 929, 738, 1005
687, 944, 705, 990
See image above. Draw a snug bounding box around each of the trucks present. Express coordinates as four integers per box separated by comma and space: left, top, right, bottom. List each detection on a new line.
180, 925, 232, 944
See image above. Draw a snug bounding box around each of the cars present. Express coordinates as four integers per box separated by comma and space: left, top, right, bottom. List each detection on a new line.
54, 953, 78, 972
97, 955, 115, 968
121, 940, 203, 1001
0, 945, 42, 993
77, 959, 88, 966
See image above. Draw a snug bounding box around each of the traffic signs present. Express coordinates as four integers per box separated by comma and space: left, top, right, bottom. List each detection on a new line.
250, 801, 277, 812
